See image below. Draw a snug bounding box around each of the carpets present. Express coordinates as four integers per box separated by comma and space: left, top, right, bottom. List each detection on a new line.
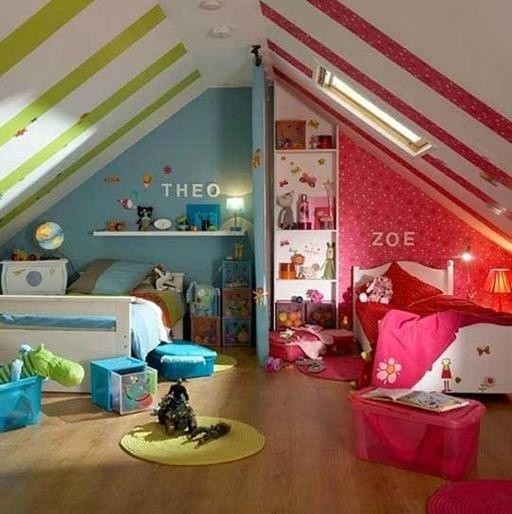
120, 416, 264, 467
296, 354, 366, 381
426, 479, 512, 513
214, 354, 237, 373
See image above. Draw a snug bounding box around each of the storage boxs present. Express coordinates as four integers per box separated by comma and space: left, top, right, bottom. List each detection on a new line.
348, 387, 486, 480
91, 356, 157, 416
269, 331, 320, 362
155, 340, 217, 381
0, 376, 41, 434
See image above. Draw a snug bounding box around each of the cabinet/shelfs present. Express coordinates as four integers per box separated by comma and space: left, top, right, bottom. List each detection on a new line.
190, 259, 252, 346
273, 120, 338, 330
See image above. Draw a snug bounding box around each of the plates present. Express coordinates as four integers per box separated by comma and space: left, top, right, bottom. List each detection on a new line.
153, 218, 172, 230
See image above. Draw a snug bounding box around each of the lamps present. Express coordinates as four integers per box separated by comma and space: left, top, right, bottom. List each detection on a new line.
482, 268, 512, 312
462, 246, 472, 261
226, 197, 245, 231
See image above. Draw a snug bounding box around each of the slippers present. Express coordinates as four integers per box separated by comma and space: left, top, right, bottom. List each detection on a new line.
295, 355, 325, 373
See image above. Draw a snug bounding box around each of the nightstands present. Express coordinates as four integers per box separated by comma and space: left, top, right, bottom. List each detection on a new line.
1, 256, 68, 296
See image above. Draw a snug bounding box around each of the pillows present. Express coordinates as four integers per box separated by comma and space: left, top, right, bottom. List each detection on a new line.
69, 258, 155, 296
356, 261, 443, 307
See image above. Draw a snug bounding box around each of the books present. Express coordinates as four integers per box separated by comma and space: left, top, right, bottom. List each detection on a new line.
361, 388, 470, 412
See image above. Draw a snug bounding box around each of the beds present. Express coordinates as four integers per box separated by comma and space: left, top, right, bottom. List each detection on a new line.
353, 260, 512, 395
0, 272, 185, 394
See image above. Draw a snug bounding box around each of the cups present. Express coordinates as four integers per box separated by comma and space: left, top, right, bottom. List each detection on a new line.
202, 220, 209, 231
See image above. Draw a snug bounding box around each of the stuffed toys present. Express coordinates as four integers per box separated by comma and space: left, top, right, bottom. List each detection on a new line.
321, 242, 335, 279
276, 191, 295, 230
135, 206, 153, 230
